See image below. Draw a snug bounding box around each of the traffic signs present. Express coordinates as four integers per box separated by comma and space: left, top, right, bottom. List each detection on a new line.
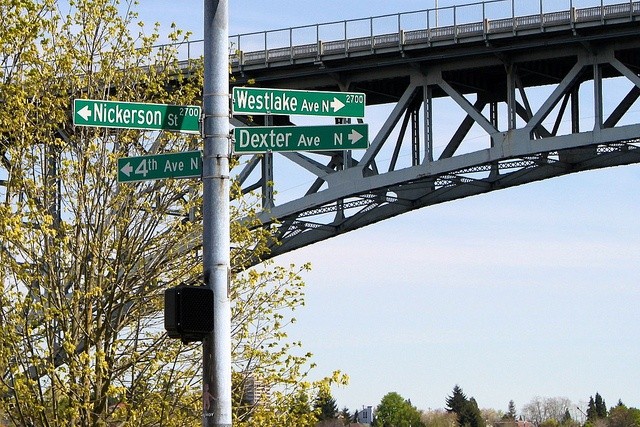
234, 124, 370, 154
72, 99, 203, 132
118, 150, 205, 182
232, 87, 366, 117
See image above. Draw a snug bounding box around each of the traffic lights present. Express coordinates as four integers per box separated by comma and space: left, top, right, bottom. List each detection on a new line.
164, 282, 214, 344
